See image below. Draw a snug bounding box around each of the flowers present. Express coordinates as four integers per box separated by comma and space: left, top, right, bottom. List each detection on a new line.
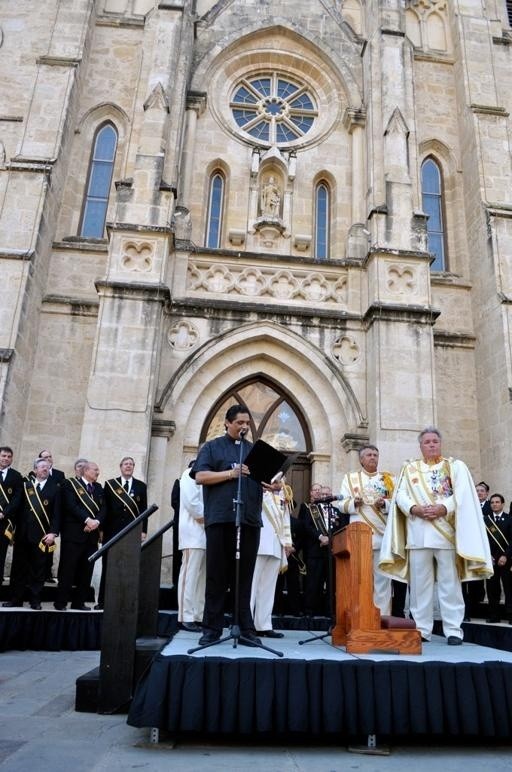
238, 426, 249, 441
311, 492, 344, 506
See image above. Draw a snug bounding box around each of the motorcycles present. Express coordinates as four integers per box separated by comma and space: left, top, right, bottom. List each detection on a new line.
186, 446, 284, 661
299, 510, 335, 648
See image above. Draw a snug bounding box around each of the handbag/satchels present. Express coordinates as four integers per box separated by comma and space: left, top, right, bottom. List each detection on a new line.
224, 467, 232, 481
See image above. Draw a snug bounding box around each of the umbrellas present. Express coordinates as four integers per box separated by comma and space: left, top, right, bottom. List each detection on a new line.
180, 622, 200, 631
238, 634, 261, 647
2, 602, 102, 610
447, 636, 462, 645
199, 636, 220, 645
258, 631, 283, 638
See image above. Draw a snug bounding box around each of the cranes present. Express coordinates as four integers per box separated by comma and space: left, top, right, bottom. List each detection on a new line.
243, 439, 301, 487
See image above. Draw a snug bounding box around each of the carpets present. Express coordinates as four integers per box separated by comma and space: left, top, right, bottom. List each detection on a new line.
495, 516, 499, 524
0, 471, 3, 481
124, 481, 128, 492
36, 483, 40, 491
88, 483, 92, 497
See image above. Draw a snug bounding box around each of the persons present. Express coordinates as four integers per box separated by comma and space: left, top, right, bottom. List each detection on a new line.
0, 445, 149, 612
260, 174, 281, 219
337, 444, 399, 622
170, 440, 351, 639
373, 426, 496, 647
390, 481, 512, 626
188, 404, 263, 649
